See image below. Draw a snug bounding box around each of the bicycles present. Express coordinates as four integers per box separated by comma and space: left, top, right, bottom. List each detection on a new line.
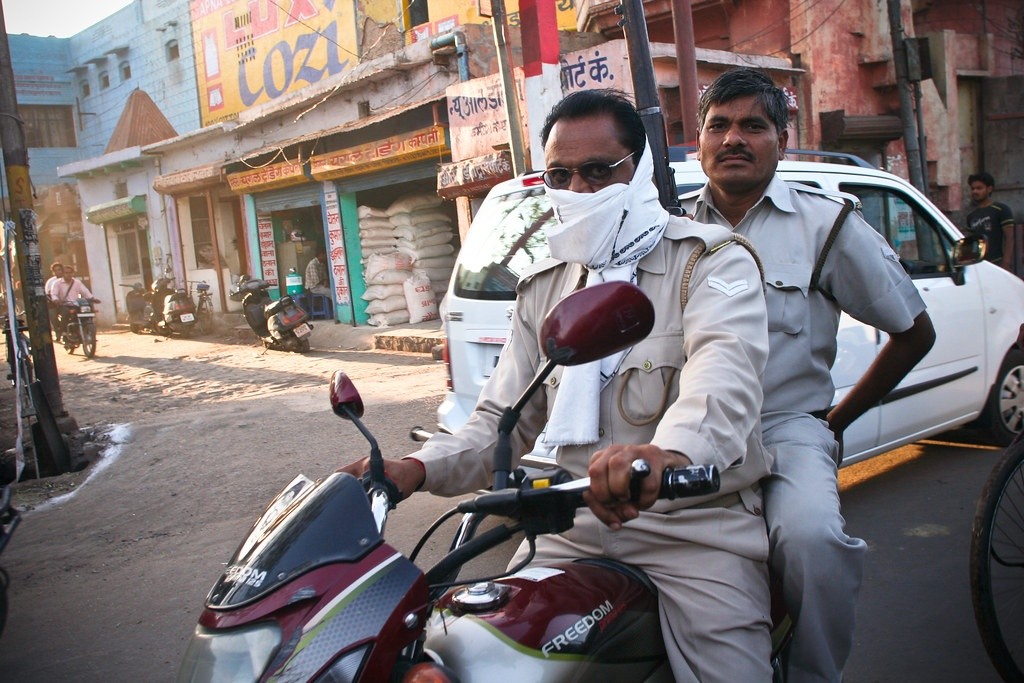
0, 310, 34, 387
180, 279, 213, 335
969, 430, 1024, 683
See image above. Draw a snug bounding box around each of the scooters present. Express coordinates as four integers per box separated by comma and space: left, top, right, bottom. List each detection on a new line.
229, 275, 314, 355
119, 276, 198, 336
55, 298, 101, 358
194, 280, 796, 683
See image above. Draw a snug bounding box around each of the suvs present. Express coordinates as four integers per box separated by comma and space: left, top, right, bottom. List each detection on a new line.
437, 147, 1024, 468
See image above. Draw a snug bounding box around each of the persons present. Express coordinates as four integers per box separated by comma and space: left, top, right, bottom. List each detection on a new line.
335, 87, 774, 683
968, 173, 1015, 272
305, 249, 332, 300
957, 239, 979, 265
688, 71, 937, 683
14, 281, 24, 319
45, 262, 100, 341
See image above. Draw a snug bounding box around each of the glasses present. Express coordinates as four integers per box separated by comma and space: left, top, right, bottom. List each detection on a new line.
538, 150, 639, 190
